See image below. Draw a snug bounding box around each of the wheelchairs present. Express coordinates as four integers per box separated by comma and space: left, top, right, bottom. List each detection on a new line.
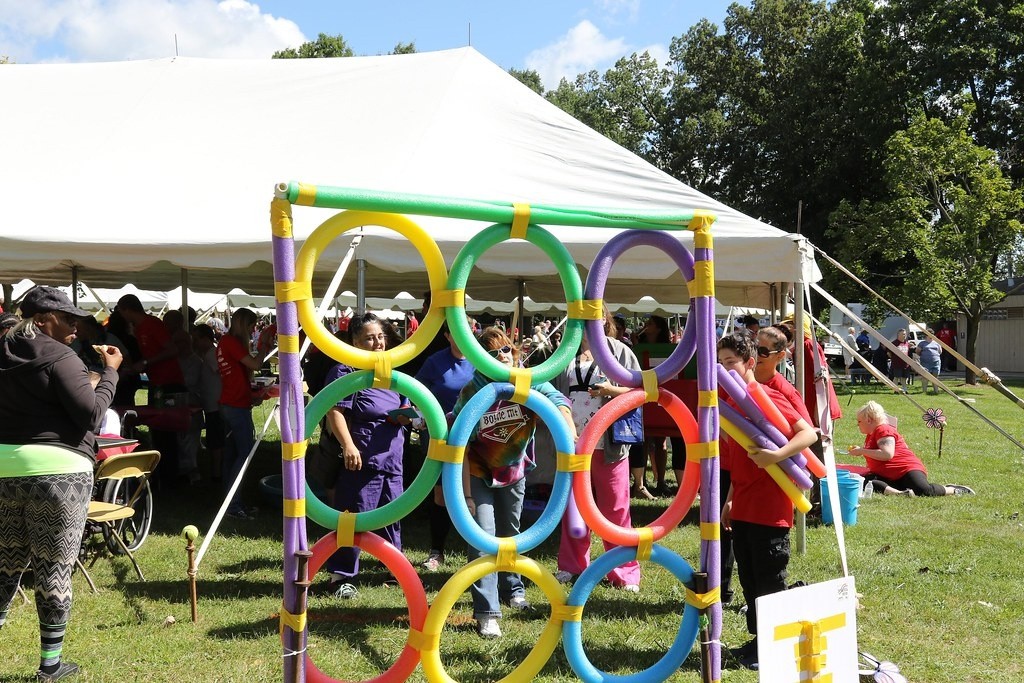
86, 410, 153, 556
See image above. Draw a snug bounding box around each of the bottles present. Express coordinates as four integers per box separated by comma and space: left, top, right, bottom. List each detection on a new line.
863, 481, 873, 499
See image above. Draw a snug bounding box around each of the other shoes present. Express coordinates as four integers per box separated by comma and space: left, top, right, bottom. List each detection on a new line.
658, 484, 672, 495
328, 577, 356, 598
382, 575, 396, 583
630, 484, 657, 501
556, 569, 574, 583
622, 585, 639, 593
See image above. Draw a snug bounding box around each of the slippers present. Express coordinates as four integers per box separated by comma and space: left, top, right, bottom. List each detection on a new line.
903, 489, 915, 498
946, 483, 975, 496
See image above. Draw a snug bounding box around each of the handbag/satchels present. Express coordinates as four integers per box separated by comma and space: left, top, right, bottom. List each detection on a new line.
308, 426, 345, 489
603, 403, 642, 442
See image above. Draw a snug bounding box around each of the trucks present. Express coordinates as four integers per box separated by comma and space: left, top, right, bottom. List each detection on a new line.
827, 302, 927, 372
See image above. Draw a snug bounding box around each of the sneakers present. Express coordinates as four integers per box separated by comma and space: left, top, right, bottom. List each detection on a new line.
475, 615, 500, 636
509, 596, 530, 613
730, 637, 759, 670
224, 507, 253, 522
37, 661, 78, 681
241, 504, 259, 516
420, 552, 444, 572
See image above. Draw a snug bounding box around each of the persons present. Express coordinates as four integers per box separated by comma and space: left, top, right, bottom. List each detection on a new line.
1, 284, 974, 678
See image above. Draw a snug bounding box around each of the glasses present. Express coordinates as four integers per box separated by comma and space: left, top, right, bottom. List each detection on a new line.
756, 346, 781, 358
489, 345, 511, 359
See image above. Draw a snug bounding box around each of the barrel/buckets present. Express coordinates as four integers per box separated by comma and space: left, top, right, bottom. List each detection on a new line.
836, 469, 851, 477
819, 477, 861, 527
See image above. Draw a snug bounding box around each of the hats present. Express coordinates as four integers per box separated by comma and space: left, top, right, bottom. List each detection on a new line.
20, 286, 92, 317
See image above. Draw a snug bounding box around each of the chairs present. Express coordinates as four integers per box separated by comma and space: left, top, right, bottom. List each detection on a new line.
75, 450, 161, 594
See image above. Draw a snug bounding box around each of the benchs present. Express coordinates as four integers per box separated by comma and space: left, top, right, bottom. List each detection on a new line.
848, 368, 915, 385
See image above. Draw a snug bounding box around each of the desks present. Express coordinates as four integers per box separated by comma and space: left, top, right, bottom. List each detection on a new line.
111, 403, 202, 432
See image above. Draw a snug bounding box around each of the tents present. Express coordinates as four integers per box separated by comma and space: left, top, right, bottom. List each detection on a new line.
0, 47, 825, 554
